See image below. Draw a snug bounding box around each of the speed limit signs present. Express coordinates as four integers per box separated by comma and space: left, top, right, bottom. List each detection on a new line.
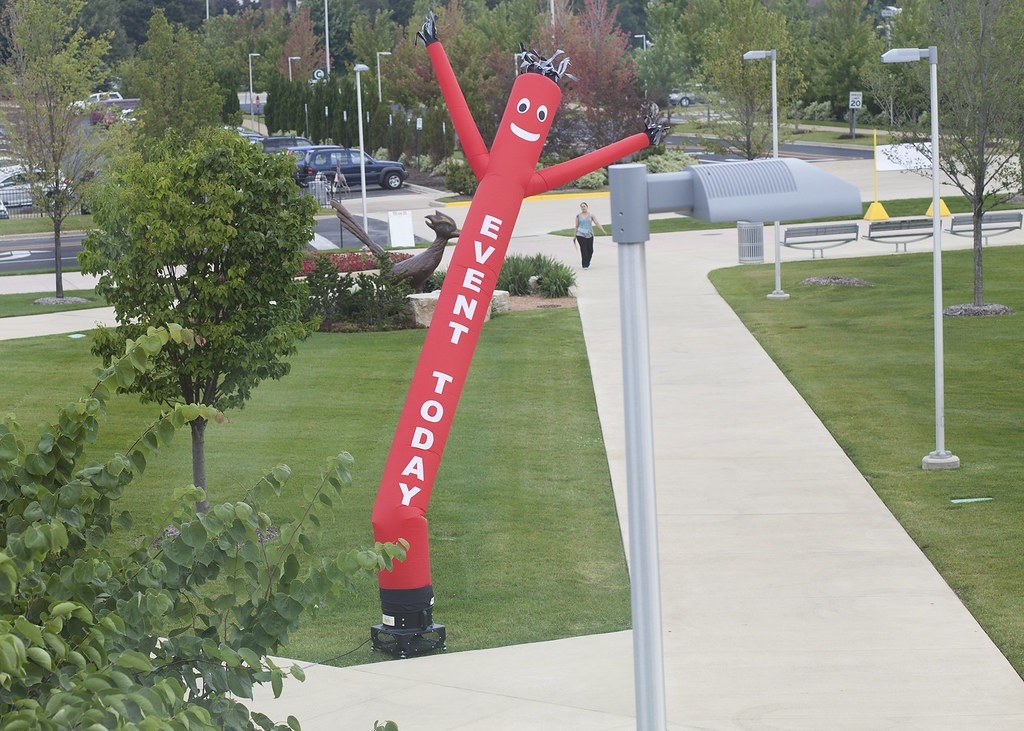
848, 91, 863, 109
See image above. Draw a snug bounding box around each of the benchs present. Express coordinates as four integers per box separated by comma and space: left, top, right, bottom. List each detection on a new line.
943, 211, 1023, 246
781, 222, 859, 258
862, 218, 943, 251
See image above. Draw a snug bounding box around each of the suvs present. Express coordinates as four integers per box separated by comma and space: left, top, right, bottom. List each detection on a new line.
303, 148, 410, 190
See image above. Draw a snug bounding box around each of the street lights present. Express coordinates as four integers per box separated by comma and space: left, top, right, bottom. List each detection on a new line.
377, 52, 391, 103
742, 48, 790, 300
352, 64, 370, 235
634, 34, 647, 100
880, 45, 961, 471
288, 56, 301, 82
249, 53, 261, 130
608, 158, 863, 731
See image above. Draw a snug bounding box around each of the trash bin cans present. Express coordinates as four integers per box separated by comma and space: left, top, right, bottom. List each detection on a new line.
736, 221, 765, 265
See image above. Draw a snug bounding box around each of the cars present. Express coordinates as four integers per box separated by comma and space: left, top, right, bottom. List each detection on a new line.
0, 149, 104, 219
241, 131, 355, 187
668, 83, 721, 107
66, 91, 141, 129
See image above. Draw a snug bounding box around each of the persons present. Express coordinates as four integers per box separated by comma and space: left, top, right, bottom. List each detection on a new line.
573, 201, 608, 270
